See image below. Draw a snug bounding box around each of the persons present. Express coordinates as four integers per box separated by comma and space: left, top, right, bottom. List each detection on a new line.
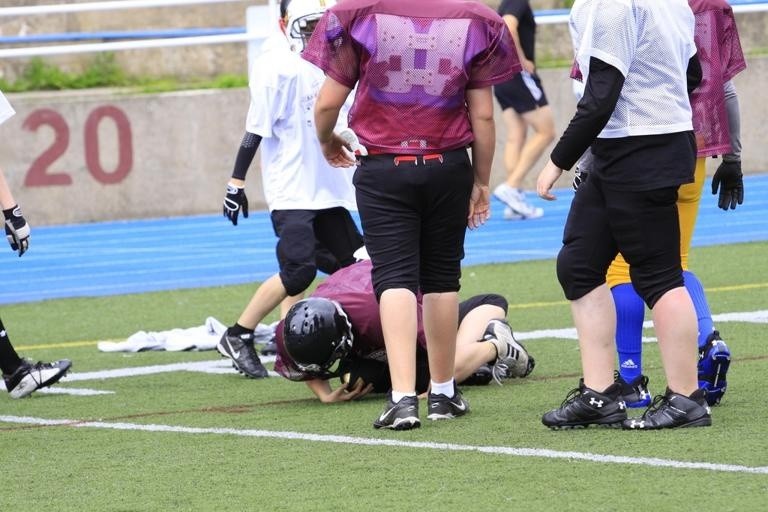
0, 91, 72, 401
217, 3, 556, 430
569, 0, 746, 407
535, 0, 712, 431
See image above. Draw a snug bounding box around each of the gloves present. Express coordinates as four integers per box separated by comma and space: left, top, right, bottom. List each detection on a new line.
221, 182, 249, 226
2, 205, 31, 257
711, 159, 745, 210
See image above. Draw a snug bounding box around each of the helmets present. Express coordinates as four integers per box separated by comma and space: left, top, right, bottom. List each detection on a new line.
273, 297, 355, 380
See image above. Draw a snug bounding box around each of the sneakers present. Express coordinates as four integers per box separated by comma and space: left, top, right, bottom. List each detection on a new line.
542, 377, 630, 429
482, 318, 537, 379
426, 386, 469, 422
462, 365, 492, 387
622, 385, 713, 431
2, 357, 74, 400
492, 182, 544, 220
698, 331, 731, 408
373, 392, 421, 431
612, 369, 654, 409
215, 324, 279, 380
